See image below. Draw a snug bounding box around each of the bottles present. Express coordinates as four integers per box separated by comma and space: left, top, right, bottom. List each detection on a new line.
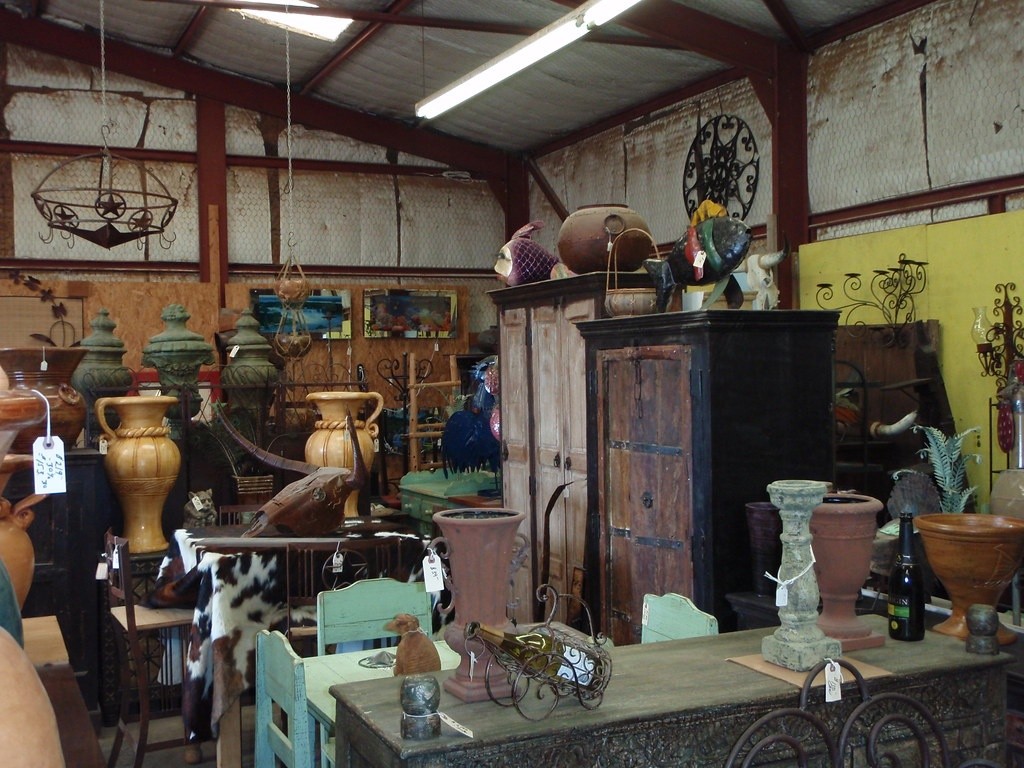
889, 513, 925, 641
468, 621, 603, 692
972, 306, 994, 344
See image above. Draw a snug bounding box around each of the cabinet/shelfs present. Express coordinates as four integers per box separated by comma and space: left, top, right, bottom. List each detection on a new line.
722, 591, 1024, 768
0, 445, 107, 740
572, 309, 843, 650
485, 271, 683, 637
100, 547, 275, 727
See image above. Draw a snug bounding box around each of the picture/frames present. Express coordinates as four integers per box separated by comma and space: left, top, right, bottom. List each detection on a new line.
249, 287, 353, 341
127, 370, 222, 431
362, 288, 458, 340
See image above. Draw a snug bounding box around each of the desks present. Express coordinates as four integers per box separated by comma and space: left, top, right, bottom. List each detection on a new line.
142, 516, 420, 768
19, 615, 105, 767
397, 467, 503, 537
328, 613, 1015, 767
300, 622, 592, 768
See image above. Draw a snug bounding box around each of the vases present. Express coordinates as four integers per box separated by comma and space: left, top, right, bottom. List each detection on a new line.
304, 392, 384, 516
0, 347, 88, 453
433, 509, 528, 703
808, 494, 885, 651
916, 514, 1024, 646
94, 396, 181, 553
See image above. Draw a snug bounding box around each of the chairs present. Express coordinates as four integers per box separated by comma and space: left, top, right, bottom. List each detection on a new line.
280, 537, 402, 768
104, 527, 243, 768
254, 579, 432, 768
642, 592, 718, 645
219, 504, 266, 527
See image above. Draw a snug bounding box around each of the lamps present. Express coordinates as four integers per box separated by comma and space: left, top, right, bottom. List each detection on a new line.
971, 306, 1001, 377
413, 0, 640, 122
